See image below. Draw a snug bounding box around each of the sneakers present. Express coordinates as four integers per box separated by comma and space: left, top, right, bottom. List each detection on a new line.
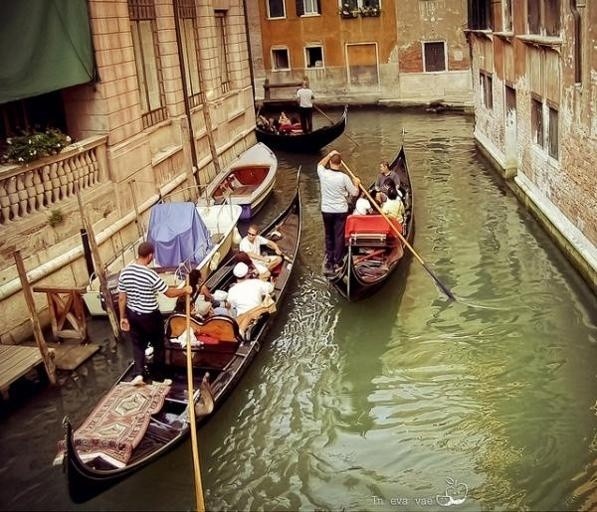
131, 375, 144, 385
152, 379, 172, 386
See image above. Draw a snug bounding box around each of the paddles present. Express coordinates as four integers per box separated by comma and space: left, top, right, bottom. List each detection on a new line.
341, 159, 455, 301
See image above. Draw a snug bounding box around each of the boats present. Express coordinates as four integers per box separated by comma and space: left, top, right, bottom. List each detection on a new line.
51, 161, 305, 510
325, 129, 419, 307
194, 139, 279, 219
307, 218, 416, 437
82, 182, 243, 321
275, 148, 324, 182
255, 99, 348, 153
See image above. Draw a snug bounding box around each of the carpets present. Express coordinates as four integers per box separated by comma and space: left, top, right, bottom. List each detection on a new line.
52, 382, 171, 468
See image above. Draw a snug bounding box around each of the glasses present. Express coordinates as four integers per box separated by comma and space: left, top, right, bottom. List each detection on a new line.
247, 231, 255, 236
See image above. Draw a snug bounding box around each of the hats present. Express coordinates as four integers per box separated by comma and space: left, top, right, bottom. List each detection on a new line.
233, 262, 248, 278
211, 290, 227, 301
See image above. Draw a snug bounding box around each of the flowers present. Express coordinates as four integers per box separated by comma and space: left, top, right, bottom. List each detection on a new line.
0, 117, 80, 168
359, 1, 382, 15
336, 0, 358, 15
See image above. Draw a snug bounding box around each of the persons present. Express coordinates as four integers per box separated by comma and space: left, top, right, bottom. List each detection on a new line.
297, 81, 315, 135
316, 150, 405, 269
116, 225, 282, 384
